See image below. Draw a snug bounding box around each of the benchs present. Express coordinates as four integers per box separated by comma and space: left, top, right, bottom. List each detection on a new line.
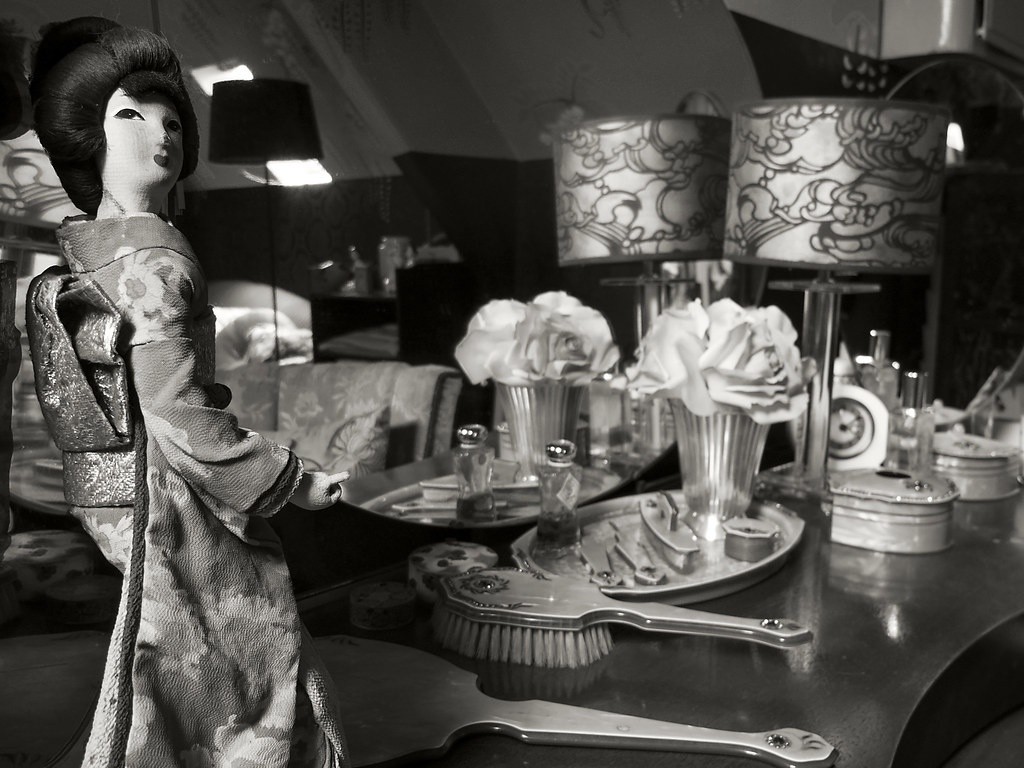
317, 261, 477, 369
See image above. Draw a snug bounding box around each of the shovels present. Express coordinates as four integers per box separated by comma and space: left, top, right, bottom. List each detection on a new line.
312, 633, 840, 768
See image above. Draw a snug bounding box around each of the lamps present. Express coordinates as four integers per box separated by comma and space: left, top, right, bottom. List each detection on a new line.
551, 114, 732, 327
206, 79, 323, 361
724, 97, 949, 496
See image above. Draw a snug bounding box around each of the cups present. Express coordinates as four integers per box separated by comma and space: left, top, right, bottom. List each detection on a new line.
379, 236, 415, 294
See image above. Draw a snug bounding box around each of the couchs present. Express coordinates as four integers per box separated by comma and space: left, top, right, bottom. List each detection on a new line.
209, 356, 467, 485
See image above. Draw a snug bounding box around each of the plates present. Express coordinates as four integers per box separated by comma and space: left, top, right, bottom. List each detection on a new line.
826, 385, 889, 471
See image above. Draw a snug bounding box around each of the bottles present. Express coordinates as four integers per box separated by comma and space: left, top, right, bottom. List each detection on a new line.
454, 425, 499, 522
539, 439, 582, 561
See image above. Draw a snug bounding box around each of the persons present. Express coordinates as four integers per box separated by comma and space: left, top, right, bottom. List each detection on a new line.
23, 18, 353, 768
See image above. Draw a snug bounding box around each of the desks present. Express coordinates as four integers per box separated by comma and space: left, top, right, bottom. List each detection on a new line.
304, 487, 1024, 768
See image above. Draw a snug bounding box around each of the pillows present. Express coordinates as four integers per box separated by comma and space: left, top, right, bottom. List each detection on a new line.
261, 405, 391, 479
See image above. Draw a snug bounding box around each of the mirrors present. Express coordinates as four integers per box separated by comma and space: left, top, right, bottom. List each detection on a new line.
77, 0, 773, 530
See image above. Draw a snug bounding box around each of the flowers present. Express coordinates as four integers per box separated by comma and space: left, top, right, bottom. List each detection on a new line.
455, 290, 621, 391
624, 298, 812, 424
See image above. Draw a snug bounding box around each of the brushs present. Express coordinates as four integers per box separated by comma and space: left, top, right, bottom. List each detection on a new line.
440, 578, 816, 664
